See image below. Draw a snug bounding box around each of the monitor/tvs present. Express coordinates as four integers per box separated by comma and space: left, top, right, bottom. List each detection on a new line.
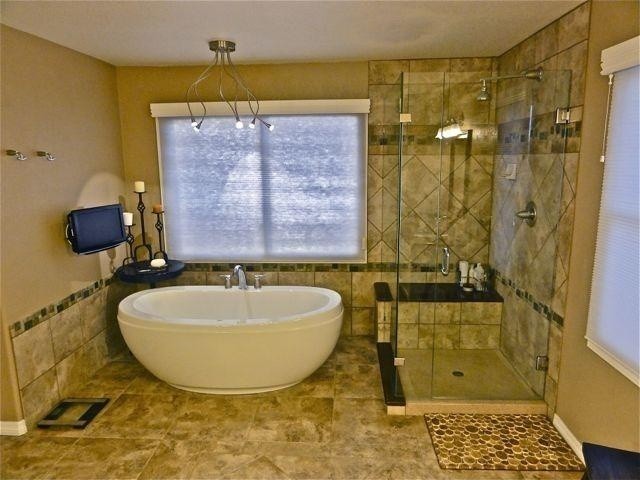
66, 203, 127, 256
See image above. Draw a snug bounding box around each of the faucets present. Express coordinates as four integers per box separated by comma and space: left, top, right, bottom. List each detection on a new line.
232, 265, 247, 289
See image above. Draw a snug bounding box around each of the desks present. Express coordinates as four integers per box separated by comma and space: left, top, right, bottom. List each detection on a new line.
582, 441, 640, 480
119, 259, 185, 289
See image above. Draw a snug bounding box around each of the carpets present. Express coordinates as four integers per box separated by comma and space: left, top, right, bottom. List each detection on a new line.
424, 412, 585, 472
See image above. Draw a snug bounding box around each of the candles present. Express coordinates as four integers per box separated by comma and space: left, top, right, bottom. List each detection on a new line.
135, 181, 145, 193
153, 205, 162, 213
123, 212, 133, 225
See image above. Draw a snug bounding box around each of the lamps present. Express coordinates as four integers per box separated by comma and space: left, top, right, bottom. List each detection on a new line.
186, 40, 273, 132
435, 111, 467, 140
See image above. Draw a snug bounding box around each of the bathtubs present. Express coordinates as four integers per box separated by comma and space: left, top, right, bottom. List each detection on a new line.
116, 286, 344, 394
